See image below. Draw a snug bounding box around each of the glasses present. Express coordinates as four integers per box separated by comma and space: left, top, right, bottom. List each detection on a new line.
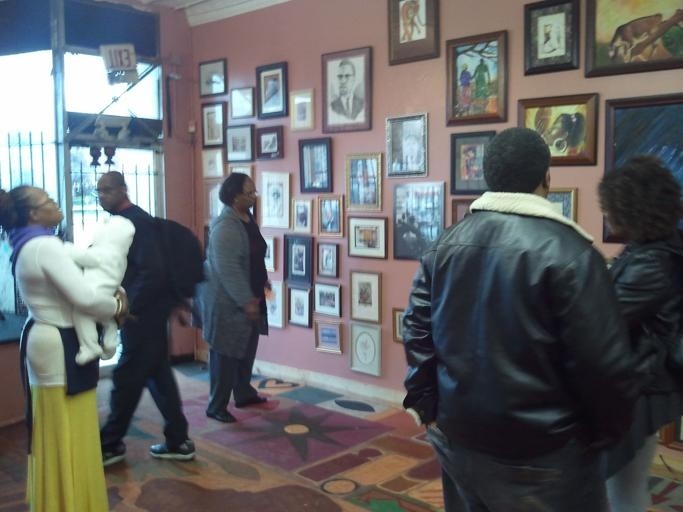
34, 198, 53, 211
241, 191, 258, 198
95, 187, 114, 192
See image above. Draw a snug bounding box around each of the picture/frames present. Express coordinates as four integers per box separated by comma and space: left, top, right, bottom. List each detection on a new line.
254, 126, 284, 161
393, 181, 447, 262
223, 124, 254, 163
201, 149, 276, 273
291, 199, 313, 234
388, 1, 440, 66
321, 47, 372, 134
347, 215, 388, 259
584, 1, 682, 78
452, 197, 479, 226
523, 2, 580, 78
445, 31, 508, 126
349, 269, 382, 325
314, 319, 344, 355
201, 102, 226, 149
516, 92, 598, 166
261, 170, 290, 228
603, 91, 682, 246
197, 58, 227, 97
311, 280, 342, 319
316, 242, 339, 278
298, 137, 333, 193
282, 233, 314, 288
345, 152, 383, 210
230, 86, 255, 120
256, 61, 288, 120
546, 186, 578, 224
391, 307, 407, 344
287, 284, 312, 328
384, 112, 428, 179
316, 194, 343, 239
286, 88, 316, 131
449, 129, 496, 194
349, 322, 382, 378
264, 281, 285, 329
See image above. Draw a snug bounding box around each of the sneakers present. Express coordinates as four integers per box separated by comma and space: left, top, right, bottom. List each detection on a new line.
103, 441, 127, 466
150, 439, 196, 460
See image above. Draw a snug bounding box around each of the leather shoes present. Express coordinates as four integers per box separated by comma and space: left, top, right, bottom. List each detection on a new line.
235, 397, 266, 407
207, 410, 235, 422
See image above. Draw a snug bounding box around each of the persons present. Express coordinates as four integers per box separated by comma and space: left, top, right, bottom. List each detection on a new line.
97, 167, 204, 466
472, 59, 491, 87
460, 64, 472, 96
331, 61, 364, 120
589, 154, 682, 511
202, 172, 268, 422
403, 127, 632, 512
0, 183, 128, 512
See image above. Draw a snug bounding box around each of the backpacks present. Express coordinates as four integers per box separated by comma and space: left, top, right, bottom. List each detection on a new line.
149, 214, 205, 327
